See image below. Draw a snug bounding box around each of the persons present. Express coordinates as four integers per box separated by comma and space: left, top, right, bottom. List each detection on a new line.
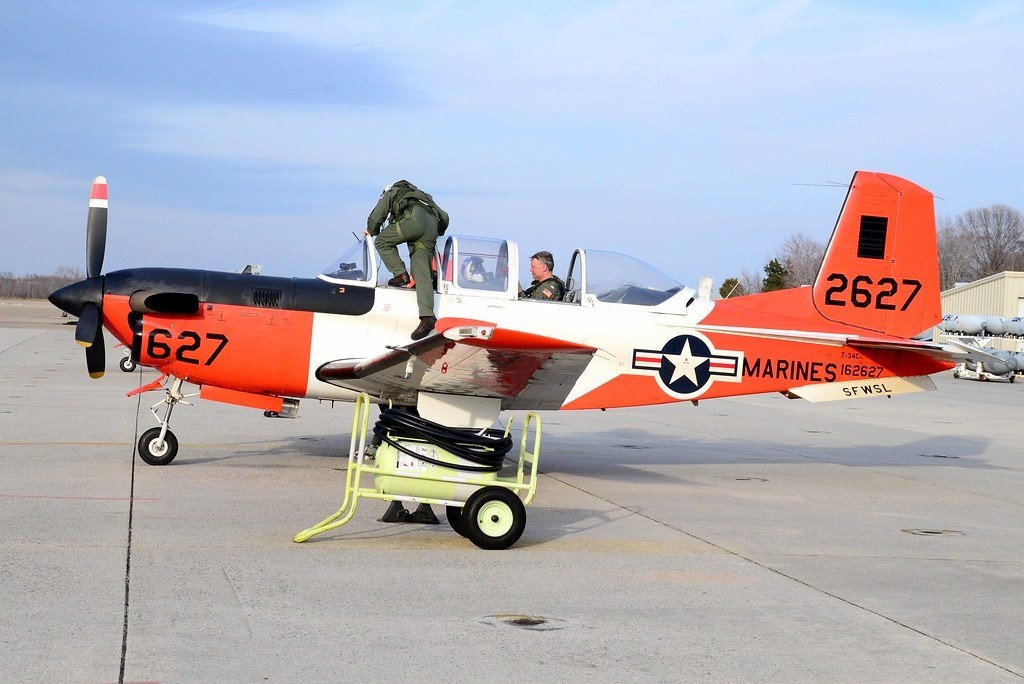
518, 251, 565, 302
362, 179, 449, 341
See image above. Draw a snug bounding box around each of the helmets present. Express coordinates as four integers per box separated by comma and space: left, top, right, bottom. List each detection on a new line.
380, 183, 393, 196
461, 256, 487, 282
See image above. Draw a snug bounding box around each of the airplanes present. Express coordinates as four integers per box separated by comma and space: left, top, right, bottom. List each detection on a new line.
935, 311, 1024, 383
48, 170, 1007, 466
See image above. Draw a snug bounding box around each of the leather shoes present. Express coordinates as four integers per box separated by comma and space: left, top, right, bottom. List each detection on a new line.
411, 316, 437, 340
388, 272, 409, 285
